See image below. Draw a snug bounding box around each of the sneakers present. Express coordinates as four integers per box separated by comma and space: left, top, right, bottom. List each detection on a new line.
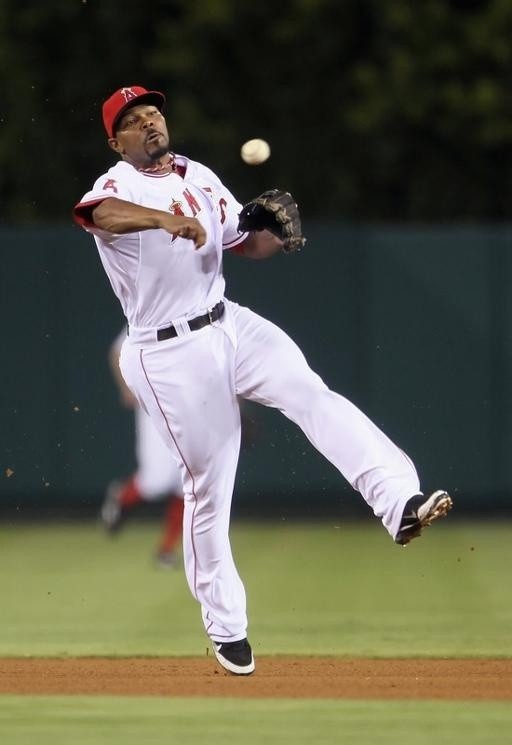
100, 480, 125, 532
213, 638, 256, 676
395, 489, 453, 546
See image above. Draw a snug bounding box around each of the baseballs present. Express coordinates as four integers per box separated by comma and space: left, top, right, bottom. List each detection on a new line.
241, 139, 271, 165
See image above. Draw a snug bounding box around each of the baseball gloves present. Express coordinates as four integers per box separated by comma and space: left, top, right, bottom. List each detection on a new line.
237, 189, 305, 254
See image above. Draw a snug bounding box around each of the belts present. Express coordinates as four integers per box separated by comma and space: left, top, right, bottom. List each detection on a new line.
158, 302, 225, 341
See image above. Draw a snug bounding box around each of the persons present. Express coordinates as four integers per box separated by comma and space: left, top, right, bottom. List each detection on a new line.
97, 323, 187, 570
69, 78, 454, 679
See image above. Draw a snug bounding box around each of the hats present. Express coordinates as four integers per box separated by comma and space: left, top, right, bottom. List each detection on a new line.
102, 86, 166, 139
